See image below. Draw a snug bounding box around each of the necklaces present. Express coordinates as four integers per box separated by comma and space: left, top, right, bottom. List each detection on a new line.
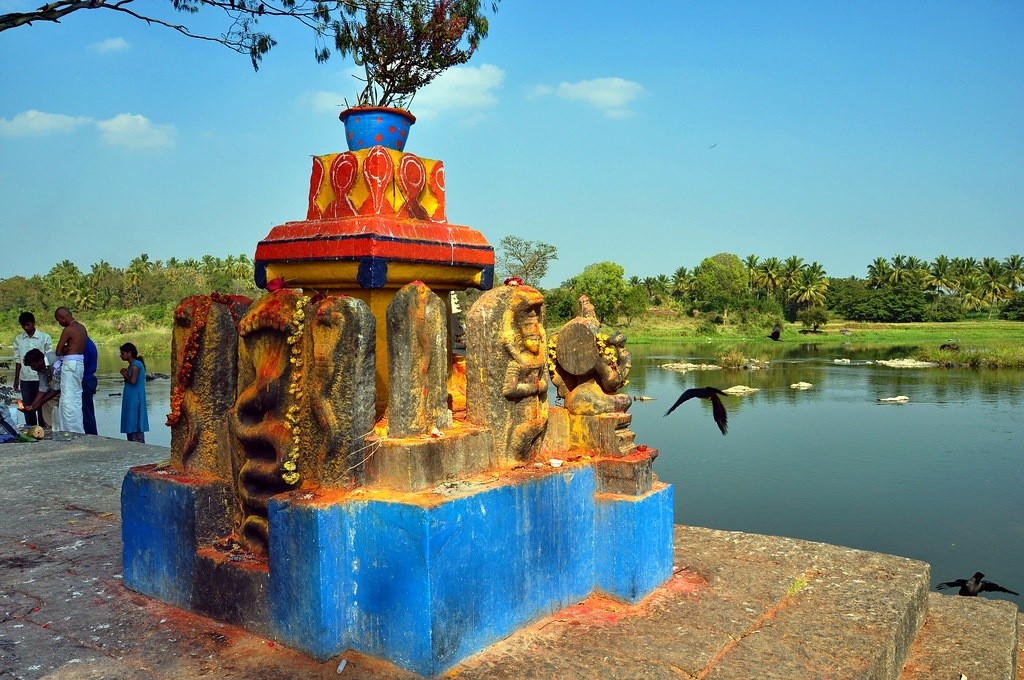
70, 319, 76, 325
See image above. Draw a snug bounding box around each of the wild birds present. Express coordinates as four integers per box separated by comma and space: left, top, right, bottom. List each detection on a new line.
663, 387, 729, 436
936, 572, 1020, 597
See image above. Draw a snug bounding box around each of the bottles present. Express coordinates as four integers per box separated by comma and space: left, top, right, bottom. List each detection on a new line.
447, 408, 454, 430
52, 406, 60, 432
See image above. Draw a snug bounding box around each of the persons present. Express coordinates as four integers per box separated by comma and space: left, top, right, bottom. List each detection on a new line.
120, 342, 149, 443
503, 313, 550, 461
82, 335, 98, 435
18, 348, 61, 429
13, 312, 52, 427
54, 306, 87, 434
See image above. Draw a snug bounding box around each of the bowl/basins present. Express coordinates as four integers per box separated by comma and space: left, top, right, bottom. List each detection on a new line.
550, 459, 563, 467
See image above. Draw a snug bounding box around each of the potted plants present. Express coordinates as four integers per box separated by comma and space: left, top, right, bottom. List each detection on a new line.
332, 0, 502, 153
